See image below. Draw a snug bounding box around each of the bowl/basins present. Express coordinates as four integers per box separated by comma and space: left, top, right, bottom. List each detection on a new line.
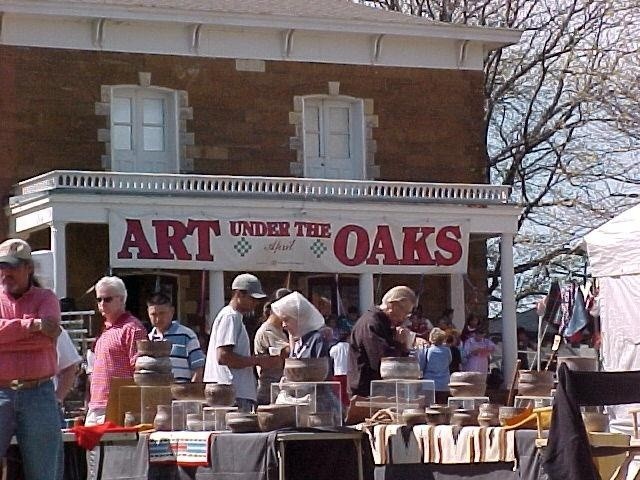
132, 339, 330, 434
378, 353, 610, 433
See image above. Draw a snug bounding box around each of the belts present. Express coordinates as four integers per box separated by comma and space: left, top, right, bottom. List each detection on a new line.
0, 373, 55, 391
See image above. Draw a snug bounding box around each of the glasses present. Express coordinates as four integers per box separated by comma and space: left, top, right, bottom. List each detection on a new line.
97, 294, 125, 303
396, 304, 414, 319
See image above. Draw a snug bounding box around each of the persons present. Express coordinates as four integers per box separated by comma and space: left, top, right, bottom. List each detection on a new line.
0, 239, 67, 480
80, 275, 151, 480
203, 269, 283, 415
255, 285, 546, 412
52, 327, 83, 404
146, 295, 206, 386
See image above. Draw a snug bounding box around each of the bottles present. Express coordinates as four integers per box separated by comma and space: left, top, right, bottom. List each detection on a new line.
63, 411, 85, 430
123, 411, 137, 427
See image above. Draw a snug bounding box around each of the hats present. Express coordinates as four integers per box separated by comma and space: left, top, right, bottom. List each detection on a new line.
0, 239, 33, 267
231, 272, 267, 300
